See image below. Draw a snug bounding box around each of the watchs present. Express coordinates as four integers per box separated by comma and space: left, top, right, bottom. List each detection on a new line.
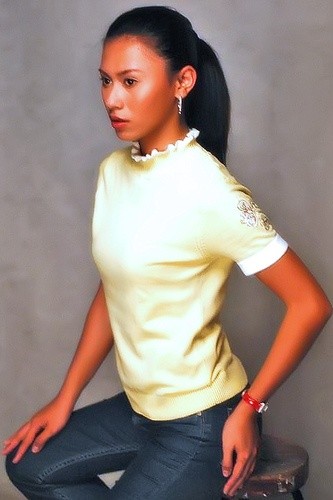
241, 389, 270, 413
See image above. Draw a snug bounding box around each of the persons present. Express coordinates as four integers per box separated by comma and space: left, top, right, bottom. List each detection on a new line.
0, 6, 333, 500
